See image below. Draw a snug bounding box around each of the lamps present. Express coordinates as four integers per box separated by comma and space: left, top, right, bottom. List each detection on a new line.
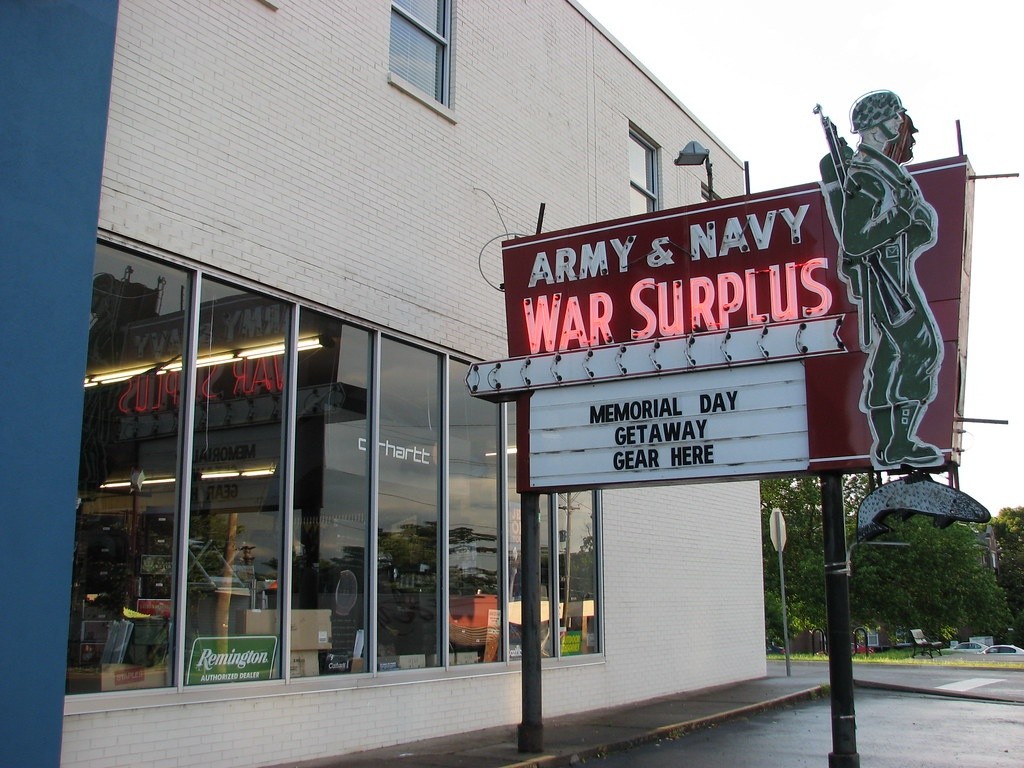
99, 459, 276, 488
674, 140, 713, 202
83, 326, 324, 388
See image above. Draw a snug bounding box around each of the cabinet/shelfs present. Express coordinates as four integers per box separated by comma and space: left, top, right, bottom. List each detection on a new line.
78, 599, 114, 666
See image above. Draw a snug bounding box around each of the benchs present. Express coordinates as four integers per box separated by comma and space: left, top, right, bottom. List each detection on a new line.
910, 629, 943, 659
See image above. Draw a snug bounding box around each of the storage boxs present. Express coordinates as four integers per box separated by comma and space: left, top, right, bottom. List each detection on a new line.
236, 608, 332, 651
426, 652, 478, 667
67, 663, 168, 692
377, 654, 425, 670
290, 649, 319, 678
125, 620, 166, 663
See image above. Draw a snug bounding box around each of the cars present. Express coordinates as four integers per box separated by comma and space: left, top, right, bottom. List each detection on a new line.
938, 642, 989, 654
849, 643, 874, 655
980, 645, 1024, 654
765, 639, 785, 655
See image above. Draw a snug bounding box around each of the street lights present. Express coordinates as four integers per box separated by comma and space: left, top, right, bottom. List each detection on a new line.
674, 139, 717, 201
